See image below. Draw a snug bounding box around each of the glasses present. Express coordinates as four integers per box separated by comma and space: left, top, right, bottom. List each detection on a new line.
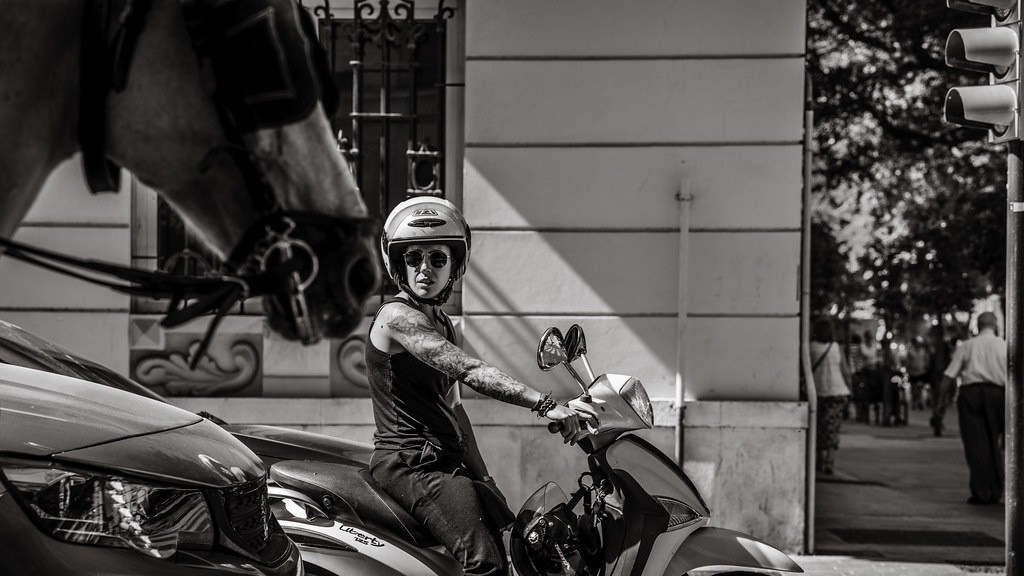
403, 250, 451, 270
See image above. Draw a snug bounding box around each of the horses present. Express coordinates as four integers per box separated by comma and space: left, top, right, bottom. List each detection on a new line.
0, 0, 379, 351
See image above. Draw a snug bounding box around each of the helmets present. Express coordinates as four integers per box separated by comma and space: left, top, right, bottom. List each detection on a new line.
380, 197, 471, 281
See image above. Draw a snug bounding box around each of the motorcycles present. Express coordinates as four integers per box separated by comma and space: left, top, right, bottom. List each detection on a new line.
266, 323, 814, 576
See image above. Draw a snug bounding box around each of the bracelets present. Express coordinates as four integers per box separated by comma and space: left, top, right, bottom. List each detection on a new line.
531, 391, 557, 418
476, 476, 496, 486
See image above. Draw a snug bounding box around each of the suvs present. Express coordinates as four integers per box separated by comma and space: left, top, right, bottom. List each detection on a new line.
0, 315, 380, 576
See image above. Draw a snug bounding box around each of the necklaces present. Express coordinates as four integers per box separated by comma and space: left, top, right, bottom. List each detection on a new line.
443, 318, 446, 338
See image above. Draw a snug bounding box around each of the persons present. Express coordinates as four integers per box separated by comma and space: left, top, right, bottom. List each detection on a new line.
365, 196, 599, 576
838, 326, 999, 437
809, 313, 853, 476
934, 312, 1007, 506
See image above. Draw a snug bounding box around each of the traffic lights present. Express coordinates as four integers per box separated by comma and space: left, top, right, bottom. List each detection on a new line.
943, 0, 1024, 143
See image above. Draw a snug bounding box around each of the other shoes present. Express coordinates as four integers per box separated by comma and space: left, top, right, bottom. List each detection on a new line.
968, 496, 992, 505
816, 455, 834, 474
930, 417, 945, 436
